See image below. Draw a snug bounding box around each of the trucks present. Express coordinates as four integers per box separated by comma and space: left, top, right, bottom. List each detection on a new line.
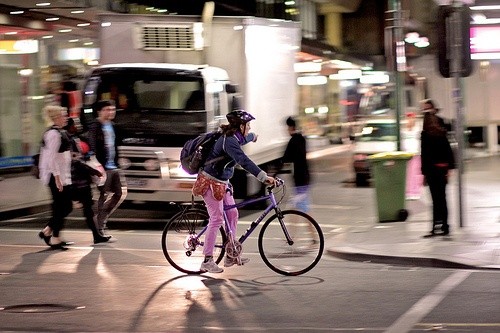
358, 55, 500, 144
77, 13, 302, 224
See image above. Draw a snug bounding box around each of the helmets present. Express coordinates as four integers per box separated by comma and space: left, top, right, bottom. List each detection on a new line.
226, 111, 256, 126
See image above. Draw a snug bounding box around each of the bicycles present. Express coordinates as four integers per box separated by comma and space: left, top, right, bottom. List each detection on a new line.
161, 177, 324, 277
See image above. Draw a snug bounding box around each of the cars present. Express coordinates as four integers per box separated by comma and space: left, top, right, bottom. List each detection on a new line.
348, 119, 417, 186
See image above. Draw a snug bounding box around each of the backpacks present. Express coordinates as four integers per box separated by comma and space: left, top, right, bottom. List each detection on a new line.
180, 130, 223, 175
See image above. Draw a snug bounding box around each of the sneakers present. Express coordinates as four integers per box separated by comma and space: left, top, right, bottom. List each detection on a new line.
224, 254, 250, 267
200, 258, 224, 273
93, 213, 106, 235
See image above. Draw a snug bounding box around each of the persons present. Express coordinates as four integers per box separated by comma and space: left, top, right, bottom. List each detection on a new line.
63, 117, 112, 245
30, 74, 80, 179
86, 100, 127, 237
419, 99, 453, 188
419, 110, 456, 238
190, 109, 276, 273
273, 115, 320, 252
38, 104, 74, 251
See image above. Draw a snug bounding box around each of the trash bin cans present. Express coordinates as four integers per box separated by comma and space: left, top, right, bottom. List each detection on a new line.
367, 152, 416, 224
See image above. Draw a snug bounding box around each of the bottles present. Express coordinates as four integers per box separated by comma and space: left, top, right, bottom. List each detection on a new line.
190, 146, 202, 168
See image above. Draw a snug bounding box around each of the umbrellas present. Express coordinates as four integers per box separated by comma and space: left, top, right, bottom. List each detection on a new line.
189, 146, 203, 171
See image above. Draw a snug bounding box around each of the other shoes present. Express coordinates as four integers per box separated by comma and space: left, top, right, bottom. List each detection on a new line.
49, 242, 68, 250
94, 236, 111, 244
38, 230, 52, 246
